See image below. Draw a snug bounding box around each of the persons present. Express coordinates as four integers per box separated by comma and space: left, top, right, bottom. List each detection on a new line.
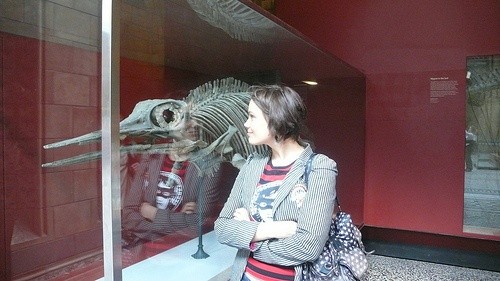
464, 130, 473, 171
214, 84, 338, 281
121, 90, 214, 263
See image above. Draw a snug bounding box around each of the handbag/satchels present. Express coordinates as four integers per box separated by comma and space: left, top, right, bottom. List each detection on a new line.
302, 153, 368, 281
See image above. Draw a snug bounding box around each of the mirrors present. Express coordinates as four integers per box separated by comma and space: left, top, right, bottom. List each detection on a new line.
462, 54, 500, 236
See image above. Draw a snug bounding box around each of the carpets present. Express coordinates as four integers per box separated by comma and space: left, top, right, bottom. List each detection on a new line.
359, 253, 500, 281
